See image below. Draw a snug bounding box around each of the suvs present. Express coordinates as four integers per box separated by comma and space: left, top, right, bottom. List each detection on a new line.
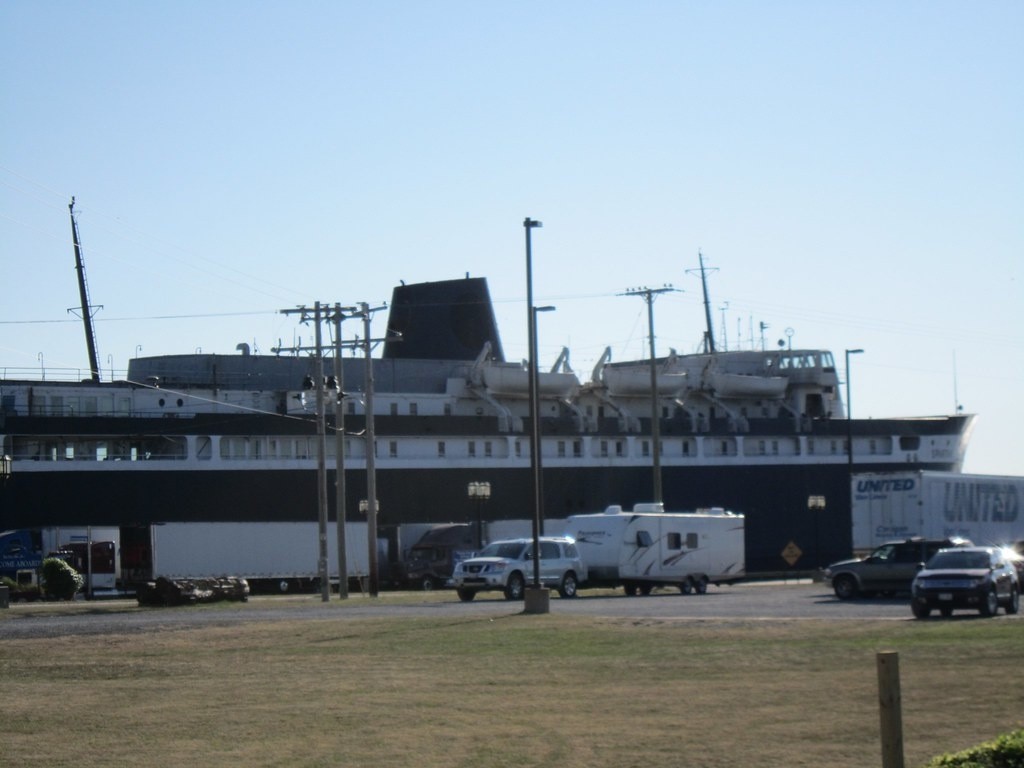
449, 536, 588, 602
827, 536, 976, 601
911, 539, 1024, 619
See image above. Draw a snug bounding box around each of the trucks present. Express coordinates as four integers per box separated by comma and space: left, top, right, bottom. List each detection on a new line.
851, 469, 1024, 556
404, 503, 746, 596
0, 521, 371, 594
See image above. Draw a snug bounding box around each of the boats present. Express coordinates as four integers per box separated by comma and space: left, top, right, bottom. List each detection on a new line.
0, 196, 969, 570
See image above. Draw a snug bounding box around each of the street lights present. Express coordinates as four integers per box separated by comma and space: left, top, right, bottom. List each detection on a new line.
468, 481, 492, 549
534, 306, 555, 537
846, 349, 864, 472
524, 217, 550, 614
